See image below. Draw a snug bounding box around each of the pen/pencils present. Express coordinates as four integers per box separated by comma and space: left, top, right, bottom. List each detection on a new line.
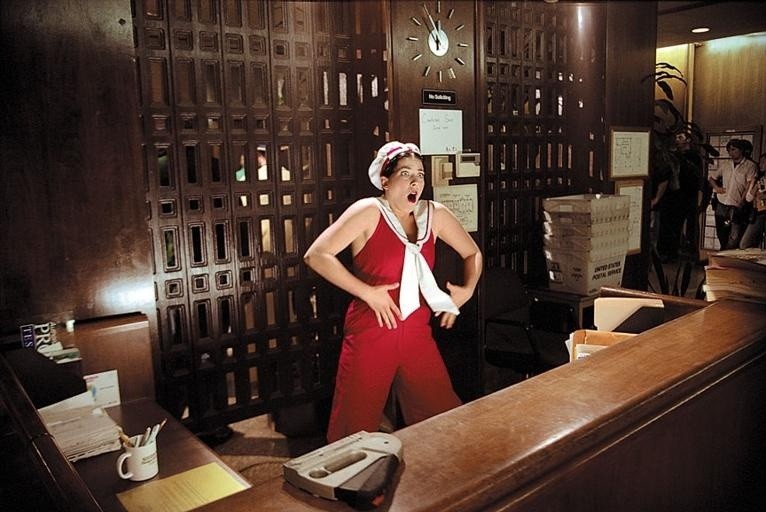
118, 417, 168, 448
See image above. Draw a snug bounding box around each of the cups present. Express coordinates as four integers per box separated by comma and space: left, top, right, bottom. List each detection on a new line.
115, 435, 160, 482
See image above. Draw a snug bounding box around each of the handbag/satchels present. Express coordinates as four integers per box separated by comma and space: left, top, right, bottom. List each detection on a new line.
745, 209, 757, 224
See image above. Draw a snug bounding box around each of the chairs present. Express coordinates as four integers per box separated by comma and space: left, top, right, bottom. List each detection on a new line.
481, 268, 580, 379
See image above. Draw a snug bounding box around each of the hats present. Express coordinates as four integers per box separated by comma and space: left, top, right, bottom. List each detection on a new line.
368, 141, 422, 190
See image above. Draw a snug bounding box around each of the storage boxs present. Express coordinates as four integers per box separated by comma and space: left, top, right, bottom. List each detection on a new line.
543, 194, 630, 296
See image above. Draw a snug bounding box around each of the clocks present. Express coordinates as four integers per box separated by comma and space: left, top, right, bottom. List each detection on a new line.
408, 0, 468, 82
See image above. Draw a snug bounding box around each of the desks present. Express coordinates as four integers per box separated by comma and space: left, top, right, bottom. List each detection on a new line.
523, 284, 599, 329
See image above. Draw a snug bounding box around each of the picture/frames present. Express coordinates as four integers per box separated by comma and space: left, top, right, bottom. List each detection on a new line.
614, 180, 645, 256
607, 126, 652, 182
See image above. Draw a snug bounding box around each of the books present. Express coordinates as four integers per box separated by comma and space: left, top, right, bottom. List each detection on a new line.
564, 328, 640, 363
36, 389, 124, 470
701, 242, 766, 306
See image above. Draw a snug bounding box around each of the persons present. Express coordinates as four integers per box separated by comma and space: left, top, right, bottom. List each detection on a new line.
154, 109, 252, 451
234, 151, 245, 181
237, 144, 306, 398
302, 139, 485, 446
645, 128, 766, 256
